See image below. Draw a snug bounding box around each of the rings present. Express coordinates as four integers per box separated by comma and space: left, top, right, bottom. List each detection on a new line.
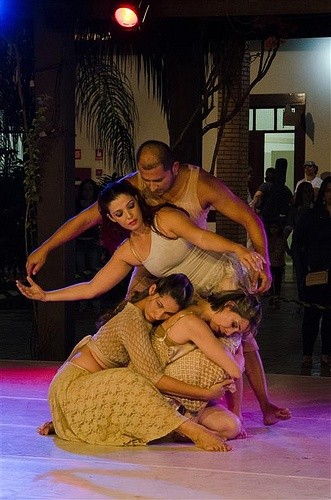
255, 256, 258, 258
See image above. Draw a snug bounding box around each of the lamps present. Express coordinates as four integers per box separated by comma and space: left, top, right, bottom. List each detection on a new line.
115, 0, 150, 30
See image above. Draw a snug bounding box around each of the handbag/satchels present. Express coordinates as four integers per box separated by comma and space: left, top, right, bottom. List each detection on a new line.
306, 271, 328, 286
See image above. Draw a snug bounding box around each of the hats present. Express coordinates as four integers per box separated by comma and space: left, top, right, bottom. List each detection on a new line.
304, 161, 318, 172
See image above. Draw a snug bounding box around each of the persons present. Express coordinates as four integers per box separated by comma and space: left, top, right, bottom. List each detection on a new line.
15, 140, 331, 452
